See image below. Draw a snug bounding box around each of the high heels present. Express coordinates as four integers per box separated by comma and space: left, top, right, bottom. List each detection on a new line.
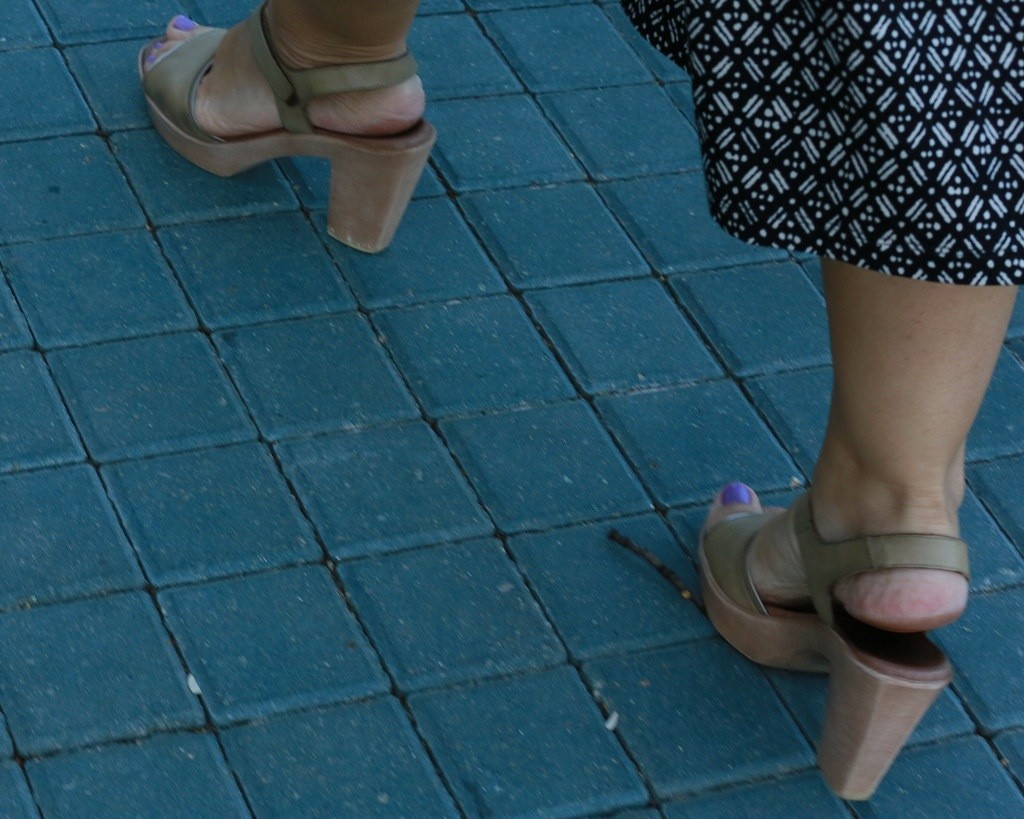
699, 487, 971, 802
136, 3, 437, 255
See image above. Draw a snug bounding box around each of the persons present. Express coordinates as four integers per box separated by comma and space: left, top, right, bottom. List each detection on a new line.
137, 0, 1024, 798
